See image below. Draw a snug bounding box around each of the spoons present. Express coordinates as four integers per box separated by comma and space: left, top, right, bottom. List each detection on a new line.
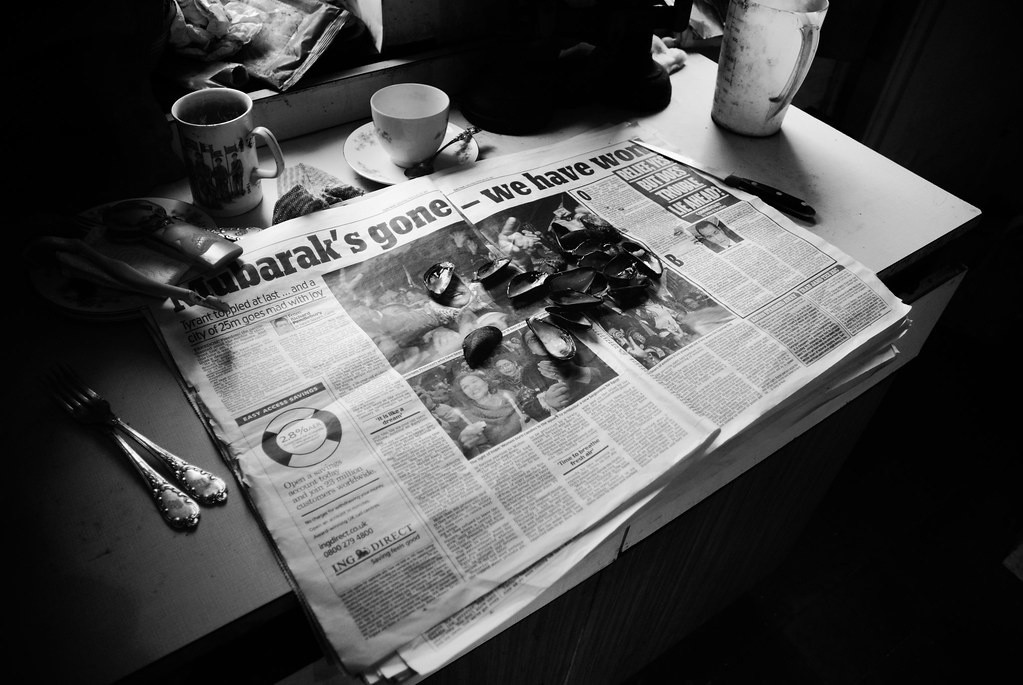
404, 125, 482, 178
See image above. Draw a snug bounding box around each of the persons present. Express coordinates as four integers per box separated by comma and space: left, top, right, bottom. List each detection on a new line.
689, 221, 745, 254
324, 190, 737, 458
271, 317, 291, 336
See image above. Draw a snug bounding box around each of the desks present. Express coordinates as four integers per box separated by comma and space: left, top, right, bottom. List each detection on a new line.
0, 55, 985, 685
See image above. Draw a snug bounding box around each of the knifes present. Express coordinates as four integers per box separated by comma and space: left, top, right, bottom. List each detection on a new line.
629, 140, 816, 222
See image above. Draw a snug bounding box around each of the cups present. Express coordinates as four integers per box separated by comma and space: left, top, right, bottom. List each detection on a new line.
370, 82, 450, 168
711, 0, 831, 136
171, 87, 285, 218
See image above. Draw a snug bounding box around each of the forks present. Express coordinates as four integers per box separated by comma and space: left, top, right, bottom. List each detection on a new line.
40, 358, 227, 530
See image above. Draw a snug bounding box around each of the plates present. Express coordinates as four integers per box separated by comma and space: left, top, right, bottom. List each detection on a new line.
343, 121, 480, 185
31, 194, 218, 322
